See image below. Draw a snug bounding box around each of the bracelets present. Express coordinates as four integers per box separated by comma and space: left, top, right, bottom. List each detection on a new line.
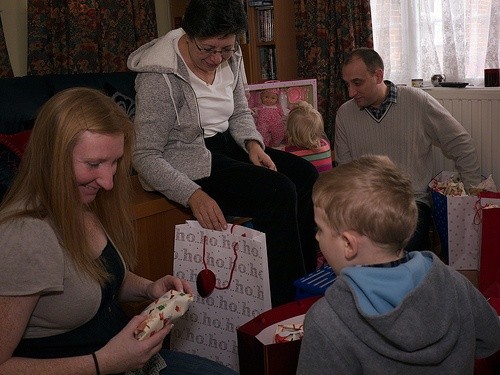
92, 352, 101, 375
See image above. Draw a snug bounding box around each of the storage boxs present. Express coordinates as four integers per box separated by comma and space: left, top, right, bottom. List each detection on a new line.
247, 79, 319, 151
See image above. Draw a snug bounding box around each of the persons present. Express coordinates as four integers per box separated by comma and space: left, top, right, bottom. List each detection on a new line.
295, 154, 500, 375
0, 88, 194, 375
126, 0, 320, 308
280, 101, 332, 176
250, 86, 286, 148
333, 47, 483, 256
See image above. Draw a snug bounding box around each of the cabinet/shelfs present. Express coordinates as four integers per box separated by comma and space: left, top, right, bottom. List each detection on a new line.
169, 0, 298, 86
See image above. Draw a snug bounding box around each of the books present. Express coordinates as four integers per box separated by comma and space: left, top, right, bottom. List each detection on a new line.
239, 0, 277, 83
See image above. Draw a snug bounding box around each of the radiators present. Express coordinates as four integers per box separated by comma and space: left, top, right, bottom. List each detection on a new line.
430, 87, 500, 193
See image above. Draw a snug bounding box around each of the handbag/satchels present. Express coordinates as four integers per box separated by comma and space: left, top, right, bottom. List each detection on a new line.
473, 190, 500, 316
170, 220, 272, 373
427, 171, 487, 271
236, 296, 324, 375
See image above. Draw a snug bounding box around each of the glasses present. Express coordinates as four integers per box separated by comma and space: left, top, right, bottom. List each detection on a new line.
192, 33, 237, 55
